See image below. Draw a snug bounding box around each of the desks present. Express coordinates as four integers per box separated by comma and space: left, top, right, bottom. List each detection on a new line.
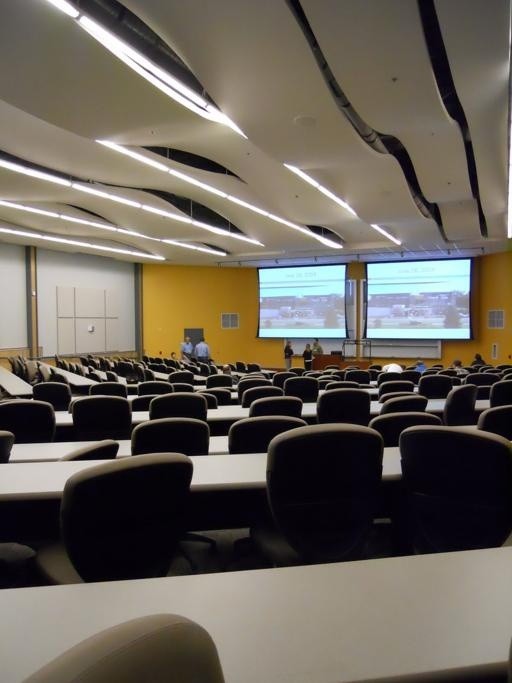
0, 546, 512, 683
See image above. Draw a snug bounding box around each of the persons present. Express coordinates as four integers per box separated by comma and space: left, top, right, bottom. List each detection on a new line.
382, 363, 403, 374
310, 338, 323, 354
470, 353, 487, 367
412, 360, 427, 376
167, 352, 178, 361
194, 337, 210, 365
451, 359, 470, 376
284, 340, 295, 368
180, 335, 193, 361
302, 343, 312, 372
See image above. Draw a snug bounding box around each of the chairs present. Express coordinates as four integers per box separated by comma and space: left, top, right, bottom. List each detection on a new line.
15, 612, 225, 683
